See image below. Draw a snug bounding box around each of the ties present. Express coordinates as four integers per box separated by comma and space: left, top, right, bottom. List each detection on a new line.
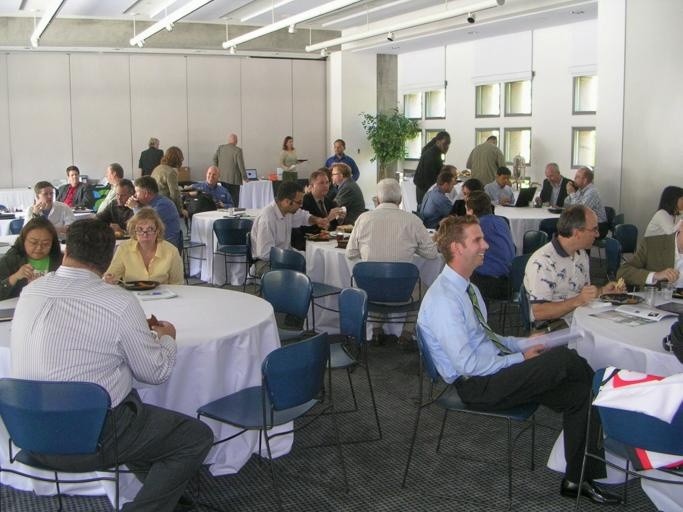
318, 200, 327, 214
465, 284, 512, 362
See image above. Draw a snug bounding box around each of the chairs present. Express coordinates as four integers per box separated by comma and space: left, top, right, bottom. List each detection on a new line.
577, 365, 678, 510
596, 207, 618, 237
350, 260, 421, 356
0, 381, 116, 512
177, 230, 206, 280
611, 223, 637, 254
194, 333, 349, 498
270, 247, 342, 332
212, 220, 251, 288
589, 238, 624, 275
8, 221, 24, 235
522, 229, 550, 249
281, 287, 386, 440
250, 270, 312, 337
499, 256, 553, 327
400, 321, 541, 508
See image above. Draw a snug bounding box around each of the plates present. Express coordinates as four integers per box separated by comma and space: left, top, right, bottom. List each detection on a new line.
124, 281, 159, 290
600, 293, 645, 304
549, 206, 566, 214
673, 289, 683, 298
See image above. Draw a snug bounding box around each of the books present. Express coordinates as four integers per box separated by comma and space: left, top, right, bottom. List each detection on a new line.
133, 285, 178, 301
589, 302, 679, 328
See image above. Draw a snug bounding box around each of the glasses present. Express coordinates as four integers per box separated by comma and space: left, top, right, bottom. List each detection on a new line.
287, 199, 302, 205
581, 225, 601, 233
331, 174, 340, 176
133, 226, 155, 233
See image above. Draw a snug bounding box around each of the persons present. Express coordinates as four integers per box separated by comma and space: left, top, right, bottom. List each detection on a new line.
345, 177, 438, 351
427, 165, 458, 206
251, 179, 330, 276
9, 219, 213, 512
0, 215, 63, 301
419, 171, 453, 233
57, 166, 94, 213
23, 181, 76, 240
484, 166, 515, 205
421, 131, 444, 155
104, 207, 185, 289
466, 135, 507, 186
330, 163, 365, 226
417, 214, 627, 505
97, 162, 123, 214
413, 131, 450, 211
564, 166, 609, 259
292, 170, 347, 250
617, 219, 683, 291
213, 134, 249, 207
644, 186, 683, 237
124, 175, 183, 257
523, 206, 627, 332
449, 178, 495, 217
536, 163, 578, 209
184, 166, 234, 215
98, 179, 135, 238
139, 138, 164, 177
280, 135, 305, 180
324, 139, 360, 181
151, 146, 183, 217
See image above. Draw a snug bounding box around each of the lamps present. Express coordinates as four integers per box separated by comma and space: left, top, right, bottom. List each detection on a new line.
386, 33, 396, 42
286, 24, 295, 34
228, 46, 237, 54
467, 14, 475, 24
163, 24, 173, 32
319, 50, 327, 59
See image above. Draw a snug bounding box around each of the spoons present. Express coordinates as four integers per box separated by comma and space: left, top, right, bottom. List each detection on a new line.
113, 278, 135, 286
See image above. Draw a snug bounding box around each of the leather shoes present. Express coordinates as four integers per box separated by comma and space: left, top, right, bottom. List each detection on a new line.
561, 478, 622, 505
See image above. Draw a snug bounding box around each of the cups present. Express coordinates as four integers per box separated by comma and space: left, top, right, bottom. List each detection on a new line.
661, 282, 673, 301
644, 287, 658, 307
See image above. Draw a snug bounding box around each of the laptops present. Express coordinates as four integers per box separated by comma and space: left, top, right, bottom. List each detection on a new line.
245, 169, 257, 181
502, 188, 536, 207
